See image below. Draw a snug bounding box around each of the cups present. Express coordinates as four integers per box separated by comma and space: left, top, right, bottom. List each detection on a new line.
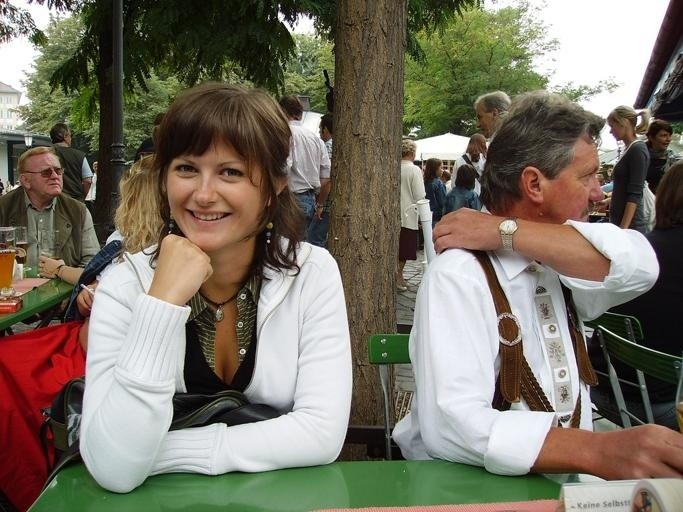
40, 230, 60, 262
0, 227, 16, 296
13, 226, 27, 264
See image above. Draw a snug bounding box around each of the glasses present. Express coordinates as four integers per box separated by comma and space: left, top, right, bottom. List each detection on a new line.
24, 168, 65, 178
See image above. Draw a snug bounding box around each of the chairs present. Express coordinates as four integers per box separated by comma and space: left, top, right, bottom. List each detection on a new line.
366, 330, 417, 463
572, 308, 651, 432
589, 321, 682, 439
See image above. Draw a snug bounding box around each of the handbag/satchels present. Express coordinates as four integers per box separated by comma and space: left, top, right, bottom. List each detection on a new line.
642, 180, 656, 233
38, 375, 281, 493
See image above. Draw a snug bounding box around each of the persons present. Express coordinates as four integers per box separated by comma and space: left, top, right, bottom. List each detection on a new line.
79, 82, 353, 494
392, 90, 683, 481
587, 160, 683, 432
279, 91, 678, 294
1, 112, 167, 512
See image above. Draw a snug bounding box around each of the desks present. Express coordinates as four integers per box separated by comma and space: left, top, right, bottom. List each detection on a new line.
18, 449, 583, 512
0, 266, 75, 339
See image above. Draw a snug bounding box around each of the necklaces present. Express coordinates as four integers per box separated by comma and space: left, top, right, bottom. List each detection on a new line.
197, 281, 250, 322
